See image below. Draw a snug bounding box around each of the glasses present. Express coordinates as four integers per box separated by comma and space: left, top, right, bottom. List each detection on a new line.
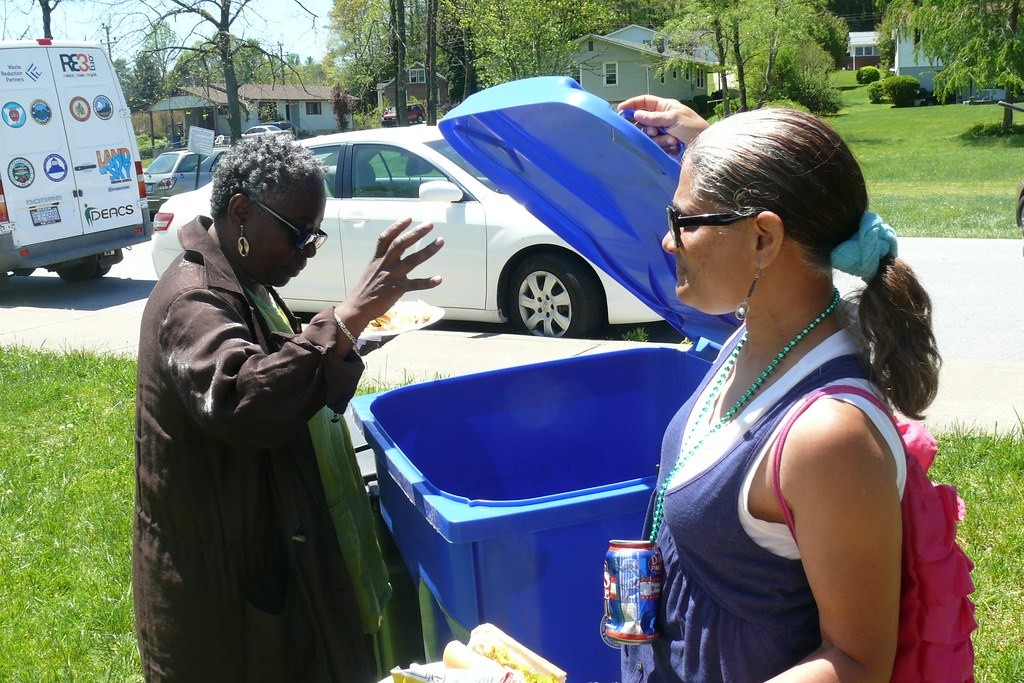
665, 204, 773, 249
248, 195, 328, 249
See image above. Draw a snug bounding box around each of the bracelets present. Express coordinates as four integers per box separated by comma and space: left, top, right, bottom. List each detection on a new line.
333, 309, 359, 346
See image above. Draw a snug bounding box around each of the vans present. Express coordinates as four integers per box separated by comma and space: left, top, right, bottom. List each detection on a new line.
0, 36, 153, 285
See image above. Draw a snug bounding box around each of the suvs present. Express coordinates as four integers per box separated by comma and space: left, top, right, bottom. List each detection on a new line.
381, 102, 427, 127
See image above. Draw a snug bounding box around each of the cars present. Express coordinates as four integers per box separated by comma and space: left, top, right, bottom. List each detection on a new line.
151, 122, 667, 340
143, 147, 230, 222
228, 123, 293, 145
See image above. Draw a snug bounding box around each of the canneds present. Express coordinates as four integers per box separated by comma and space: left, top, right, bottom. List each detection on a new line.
603, 540, 663, 645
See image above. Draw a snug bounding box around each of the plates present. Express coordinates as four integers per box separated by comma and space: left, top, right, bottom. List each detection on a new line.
358, 301, 446, 338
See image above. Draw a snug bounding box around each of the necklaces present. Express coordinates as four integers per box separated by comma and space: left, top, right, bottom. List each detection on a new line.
647, 283, 841, 544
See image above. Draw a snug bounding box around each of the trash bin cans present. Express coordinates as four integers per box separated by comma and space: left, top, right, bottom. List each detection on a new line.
343, 70, 746, 682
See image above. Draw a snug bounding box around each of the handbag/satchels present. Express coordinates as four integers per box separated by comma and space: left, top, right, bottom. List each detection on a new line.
773, 385, 978, 683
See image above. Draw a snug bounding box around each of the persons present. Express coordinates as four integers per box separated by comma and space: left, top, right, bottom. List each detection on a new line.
617, 92, 943, 683
131, 129, 446, 682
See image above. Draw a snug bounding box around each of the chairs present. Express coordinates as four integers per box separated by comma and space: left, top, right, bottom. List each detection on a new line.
405, 154, 434, 199
357, 163, 391, 198
